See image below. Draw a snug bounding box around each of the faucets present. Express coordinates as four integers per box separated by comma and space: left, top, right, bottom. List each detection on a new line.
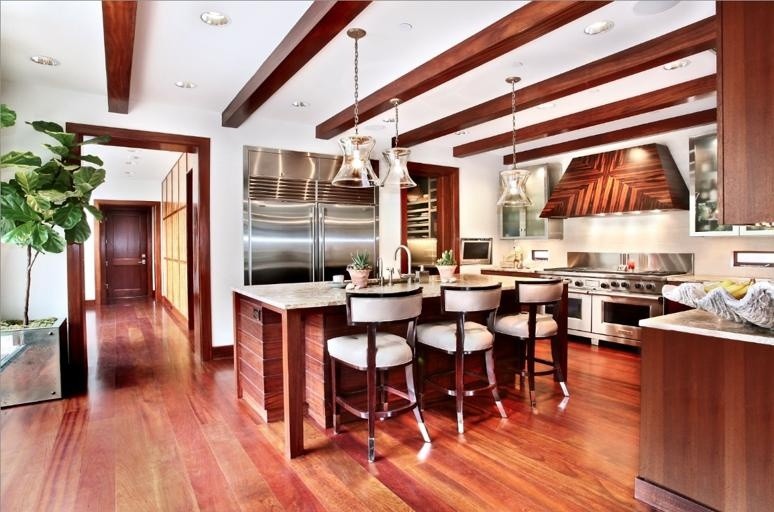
376, 256, 384, 286
395, 245, 414, 284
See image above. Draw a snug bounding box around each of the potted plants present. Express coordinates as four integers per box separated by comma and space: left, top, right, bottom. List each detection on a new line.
345, 247, 373, 289
436, 248, 458, 276
0, 102, 111, 408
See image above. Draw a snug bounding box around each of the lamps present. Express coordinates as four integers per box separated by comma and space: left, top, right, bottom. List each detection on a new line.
379, 99, 417, 189
497, 76, 535, 207
331, 29, 381, 188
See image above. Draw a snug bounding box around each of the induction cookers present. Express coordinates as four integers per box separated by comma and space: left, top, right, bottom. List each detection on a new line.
542, 265, 688, 275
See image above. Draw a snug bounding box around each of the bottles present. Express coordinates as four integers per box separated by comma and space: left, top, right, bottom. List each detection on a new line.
708, 179, 717, 201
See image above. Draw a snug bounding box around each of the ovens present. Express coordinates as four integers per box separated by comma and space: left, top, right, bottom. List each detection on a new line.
567, 274, 667, 348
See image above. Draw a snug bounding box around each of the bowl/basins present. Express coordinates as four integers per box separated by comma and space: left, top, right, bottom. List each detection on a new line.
407, 195, 419, 202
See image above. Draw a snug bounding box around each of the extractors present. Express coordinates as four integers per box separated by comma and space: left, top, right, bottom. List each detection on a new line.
539, 143, 691, 220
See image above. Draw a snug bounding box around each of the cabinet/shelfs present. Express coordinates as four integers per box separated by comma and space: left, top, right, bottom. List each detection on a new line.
635, 307, 774, 512
407, 173, 437, 266
497, 160, 563, 240
716, 0, 774, 224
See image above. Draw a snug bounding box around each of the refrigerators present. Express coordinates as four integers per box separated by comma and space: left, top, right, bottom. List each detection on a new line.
245, 200, 379, 287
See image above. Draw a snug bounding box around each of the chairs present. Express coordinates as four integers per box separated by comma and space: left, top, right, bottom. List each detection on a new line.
495, 279, 570, 408
327, 287, 431, 462
417, 282, 508, 432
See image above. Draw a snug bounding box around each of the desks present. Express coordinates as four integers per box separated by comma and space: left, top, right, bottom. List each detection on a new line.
2, 342, 27, 373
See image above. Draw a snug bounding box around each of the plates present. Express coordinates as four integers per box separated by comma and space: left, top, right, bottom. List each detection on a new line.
325, 282, 352, 287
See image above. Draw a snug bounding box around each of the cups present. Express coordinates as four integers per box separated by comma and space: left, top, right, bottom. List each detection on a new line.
333, 274, 344, 283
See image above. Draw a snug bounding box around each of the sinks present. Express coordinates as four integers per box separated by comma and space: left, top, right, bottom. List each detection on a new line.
347, 279, 419, 287
